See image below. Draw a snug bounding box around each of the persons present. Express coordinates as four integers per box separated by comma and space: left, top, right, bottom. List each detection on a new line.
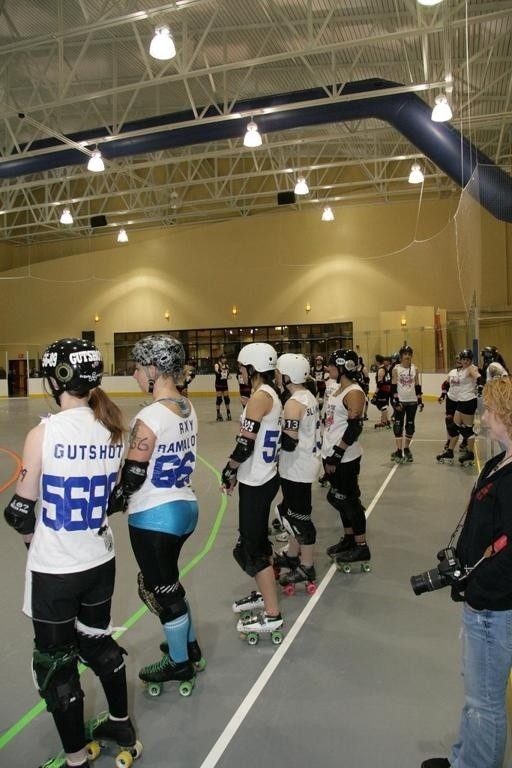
214, 351, 400, 430
220, 342, 285, 645
120, 333, 206, 696
391, 346, 424, 465
420, 375, 512, 767
321, 348, 373, 573
5, 337, 143, 766
271, 353, 320, 596
435, 346, 508, 468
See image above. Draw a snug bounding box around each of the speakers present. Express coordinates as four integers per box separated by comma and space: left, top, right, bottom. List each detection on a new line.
81, 330, 95, 342
277, 190, 297, 205
90, 215, 107, 228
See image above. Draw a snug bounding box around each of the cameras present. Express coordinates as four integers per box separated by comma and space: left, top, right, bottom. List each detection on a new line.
410, 546, 464, 596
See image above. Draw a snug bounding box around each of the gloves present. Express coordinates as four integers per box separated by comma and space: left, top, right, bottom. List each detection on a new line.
416, 398, 424, 411
438, 394, 446, 403
392, 397, 401, 407
